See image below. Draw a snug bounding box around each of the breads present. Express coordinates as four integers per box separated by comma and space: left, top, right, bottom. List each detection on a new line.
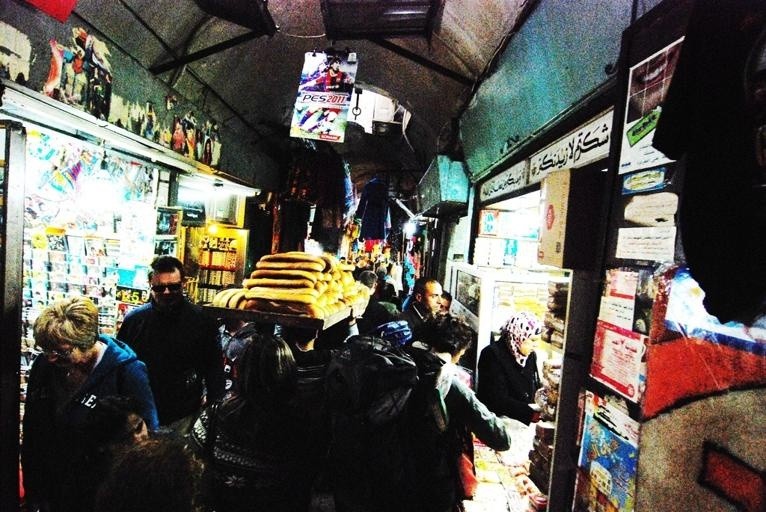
209, 248, 369, 322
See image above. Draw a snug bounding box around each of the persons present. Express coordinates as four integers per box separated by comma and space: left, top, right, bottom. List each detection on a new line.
300, 57, 354, 135
477, 311, 545, 426
0, 26, 221, 165
22, 247, 512, 512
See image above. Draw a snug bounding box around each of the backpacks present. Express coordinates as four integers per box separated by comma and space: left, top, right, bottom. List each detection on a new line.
317, 336, 453, 501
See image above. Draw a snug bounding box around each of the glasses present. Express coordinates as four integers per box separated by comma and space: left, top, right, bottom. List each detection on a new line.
34, 343, 75, 358
151, 283, 181, 292
